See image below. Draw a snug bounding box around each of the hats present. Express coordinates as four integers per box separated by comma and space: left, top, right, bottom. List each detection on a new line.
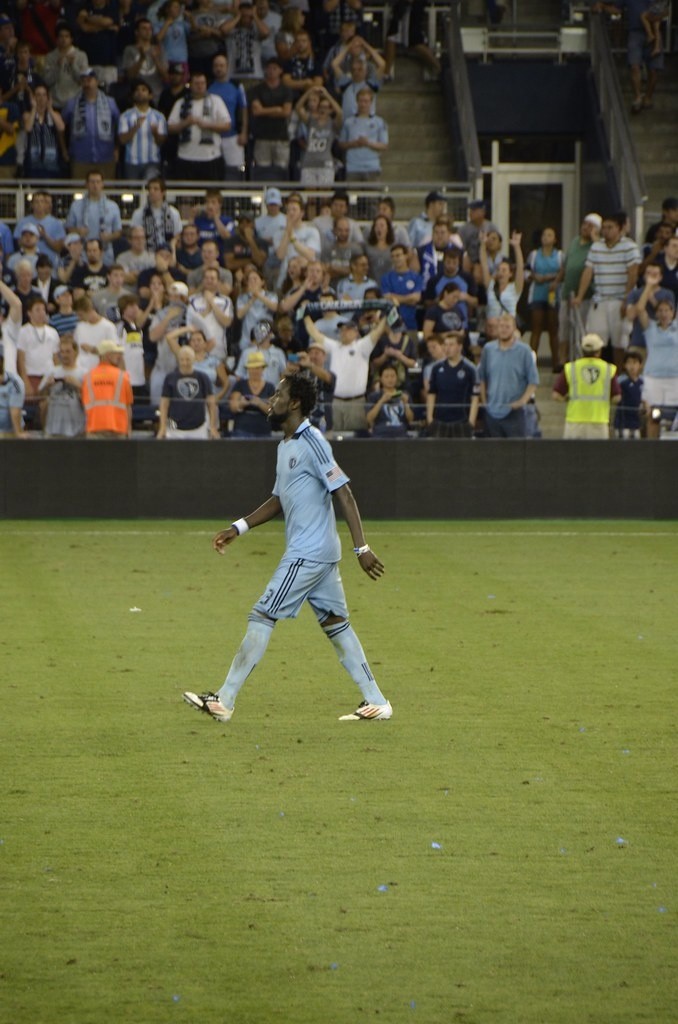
54, 285, 74, 300
170, 282, 189, 297
307, 342, 326, 354
20, 222, 39, 237
424, 191, 447, 208
466, 200, 487, 211
584, 214, 602, 229
64, 232, 83, 247
245, 352, 266, 369
96, 340, 126, 355
77, 68, 97, 80
336, 321, 358, 332
264, 189, 282, 206
582, 334, 604, 352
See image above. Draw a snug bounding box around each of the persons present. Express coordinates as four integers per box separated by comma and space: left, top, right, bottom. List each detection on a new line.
22, 82, 65, 178
167, 72, 231, 180
524, 227, 565, 370
132, 177, 182, 245
156, 345, 220, 440
81, 339, 134, 440
553, 198, 678, 439
118, 80, 166, 179
66, 170, 121, 265
38, 334, 88, 437
339, 86, 389, 182
191, 186, 235, 266
296, 85, 343, 220
254, 187, 287, 243
480, 313, 540, 437
0, 0, 508, 179
427, 331, 480, 438
592, 0, 670, 113
0, 191, 541, 440
61, 67, 121, 179
182, 368, 393, 725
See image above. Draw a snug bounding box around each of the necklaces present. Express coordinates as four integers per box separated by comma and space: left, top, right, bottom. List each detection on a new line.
33, 326, 46, 343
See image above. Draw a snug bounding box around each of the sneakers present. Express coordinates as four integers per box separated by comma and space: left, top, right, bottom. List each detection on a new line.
183, 691, 235, 723
339, 700, 393, 722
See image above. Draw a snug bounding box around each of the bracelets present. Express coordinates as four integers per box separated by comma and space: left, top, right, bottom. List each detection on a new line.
232, 518, 250, 536
354, 544, 371, 557
